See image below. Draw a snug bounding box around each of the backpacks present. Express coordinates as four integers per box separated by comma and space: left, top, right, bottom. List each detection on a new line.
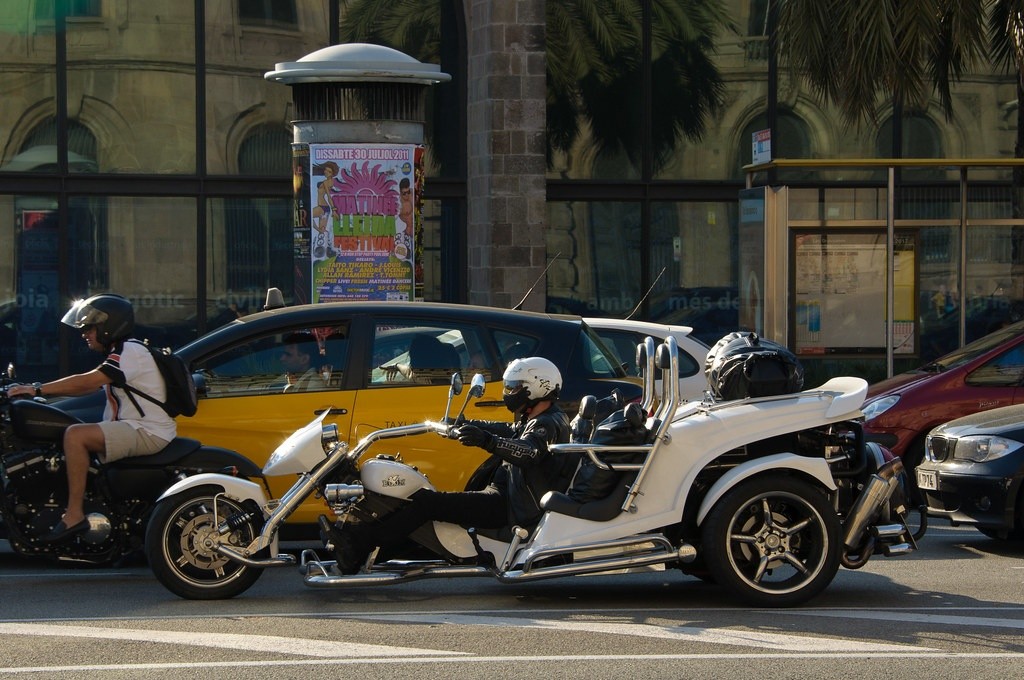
114, 341, 199, 418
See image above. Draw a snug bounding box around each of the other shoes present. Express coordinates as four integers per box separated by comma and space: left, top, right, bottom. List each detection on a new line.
44, 517, 91, 544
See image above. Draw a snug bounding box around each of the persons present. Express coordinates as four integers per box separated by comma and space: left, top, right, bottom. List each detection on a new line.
318, 357, 570, 575
381, 336, 450, 384
278, 332, 325, 391
6, 291, 175, 545
461, 349, 490, 384
922, 279, 981, 317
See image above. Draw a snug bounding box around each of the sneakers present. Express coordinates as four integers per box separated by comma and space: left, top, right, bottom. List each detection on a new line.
319, 515, 366, 573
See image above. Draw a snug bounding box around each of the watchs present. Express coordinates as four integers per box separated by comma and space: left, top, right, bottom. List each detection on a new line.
32, 380, 41, 396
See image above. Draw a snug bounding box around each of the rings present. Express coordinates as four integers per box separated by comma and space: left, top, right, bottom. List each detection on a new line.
14, 386, 18, 391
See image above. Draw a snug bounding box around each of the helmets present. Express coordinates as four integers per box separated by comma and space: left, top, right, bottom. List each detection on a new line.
60, 293, 135, 344
503, 357, 561, 411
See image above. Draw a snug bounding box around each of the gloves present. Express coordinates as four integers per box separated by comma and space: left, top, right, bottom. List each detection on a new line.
458, 423, 487, 449
440, 414, 469, 439
